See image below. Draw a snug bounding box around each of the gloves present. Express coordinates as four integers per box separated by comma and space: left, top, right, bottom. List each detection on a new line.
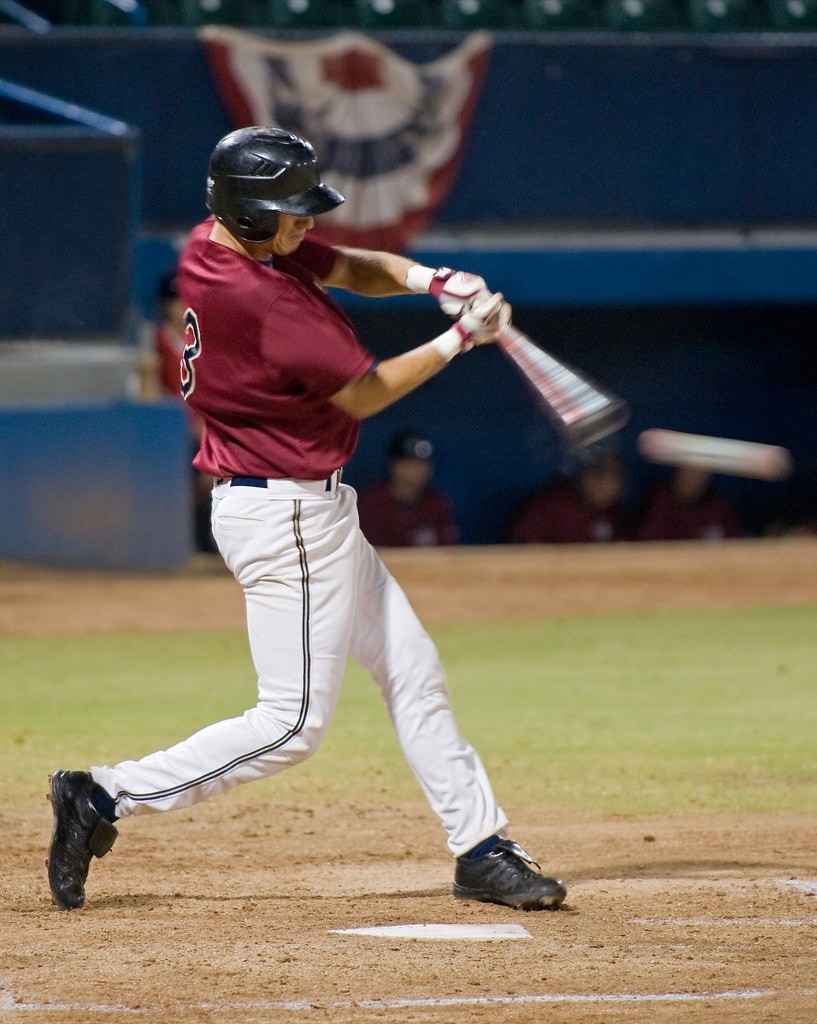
430, 266, 493, 325
450, 290, 514, 353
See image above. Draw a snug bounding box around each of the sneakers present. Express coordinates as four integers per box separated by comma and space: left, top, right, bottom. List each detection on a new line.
452, 839, 568, 911
47, 767, 120, 912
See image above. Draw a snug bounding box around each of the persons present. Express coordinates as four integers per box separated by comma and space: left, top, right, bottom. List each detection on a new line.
138, 267, 742, 547
48, 126, 567, 909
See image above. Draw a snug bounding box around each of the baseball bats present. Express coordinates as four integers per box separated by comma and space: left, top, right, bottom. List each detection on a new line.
485, 317, 628, 450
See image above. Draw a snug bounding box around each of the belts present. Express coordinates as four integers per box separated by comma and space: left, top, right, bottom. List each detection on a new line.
230, 467, 343, 491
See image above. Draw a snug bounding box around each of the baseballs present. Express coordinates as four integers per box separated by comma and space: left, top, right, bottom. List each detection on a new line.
639, 426, 786, 484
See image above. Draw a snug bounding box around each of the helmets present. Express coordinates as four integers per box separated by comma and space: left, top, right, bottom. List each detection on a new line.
206, 126, 348, 243
